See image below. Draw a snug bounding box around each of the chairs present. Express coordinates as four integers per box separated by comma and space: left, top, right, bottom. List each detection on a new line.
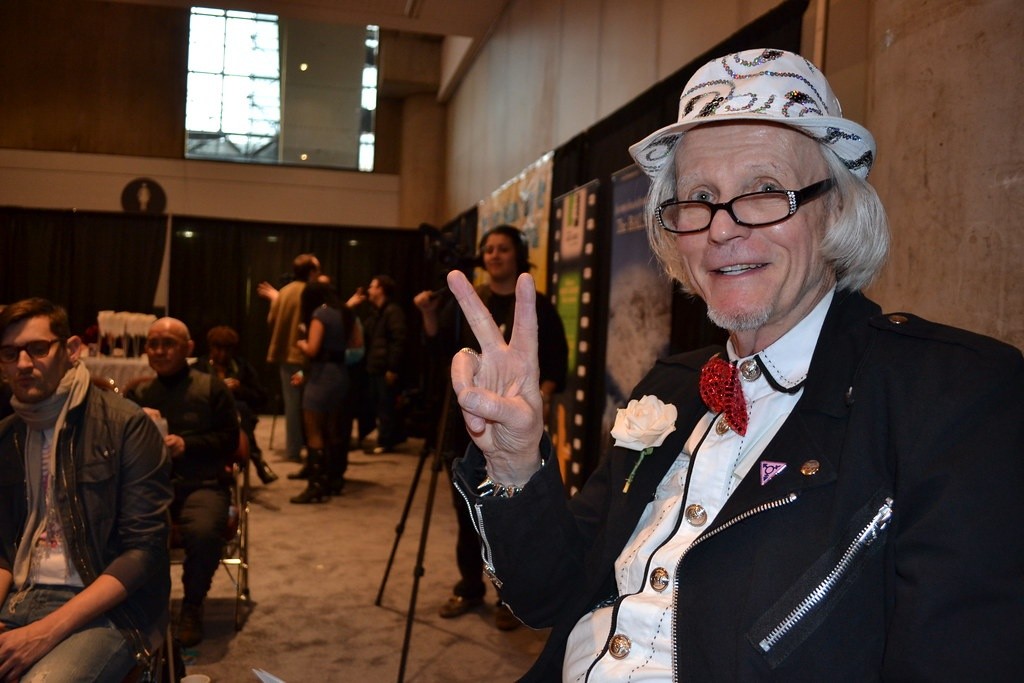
125, 430, 251, 683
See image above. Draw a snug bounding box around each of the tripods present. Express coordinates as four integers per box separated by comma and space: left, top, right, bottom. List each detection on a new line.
375, 287, 471, 683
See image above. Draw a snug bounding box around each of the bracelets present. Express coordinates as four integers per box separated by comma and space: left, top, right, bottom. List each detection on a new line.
540, 390, 554, 405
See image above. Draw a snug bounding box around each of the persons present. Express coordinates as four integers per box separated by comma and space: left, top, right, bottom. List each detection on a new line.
0, 306, 279, 647
414, 224, 568, 631
0, 298, 174, 683
258, 255, 406, 504
448, 48, 1024, 683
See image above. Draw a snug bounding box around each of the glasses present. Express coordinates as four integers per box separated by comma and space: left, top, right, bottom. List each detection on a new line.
655, 177, 838, 234
0, 338, 61, 363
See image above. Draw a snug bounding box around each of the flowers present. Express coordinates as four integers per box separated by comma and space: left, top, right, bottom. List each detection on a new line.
611, 394, 679, 493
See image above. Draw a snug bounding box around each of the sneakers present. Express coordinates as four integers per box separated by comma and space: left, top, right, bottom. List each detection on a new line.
176, 596, 204, 647
496, 605, 522, 631
258, 462, 278, 485
439, 593, 484, 618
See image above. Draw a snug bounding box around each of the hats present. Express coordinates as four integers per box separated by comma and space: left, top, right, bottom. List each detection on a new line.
207, 326, 238, 349
626, 48, 877, 185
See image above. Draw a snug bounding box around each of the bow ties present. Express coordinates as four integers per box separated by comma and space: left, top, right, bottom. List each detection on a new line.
699, 356, 748, 436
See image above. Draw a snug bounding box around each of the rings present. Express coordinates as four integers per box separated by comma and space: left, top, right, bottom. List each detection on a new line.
461, 347, 482, 375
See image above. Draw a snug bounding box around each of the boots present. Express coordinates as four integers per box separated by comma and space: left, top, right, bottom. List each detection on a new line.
287, 446, 324, 504
